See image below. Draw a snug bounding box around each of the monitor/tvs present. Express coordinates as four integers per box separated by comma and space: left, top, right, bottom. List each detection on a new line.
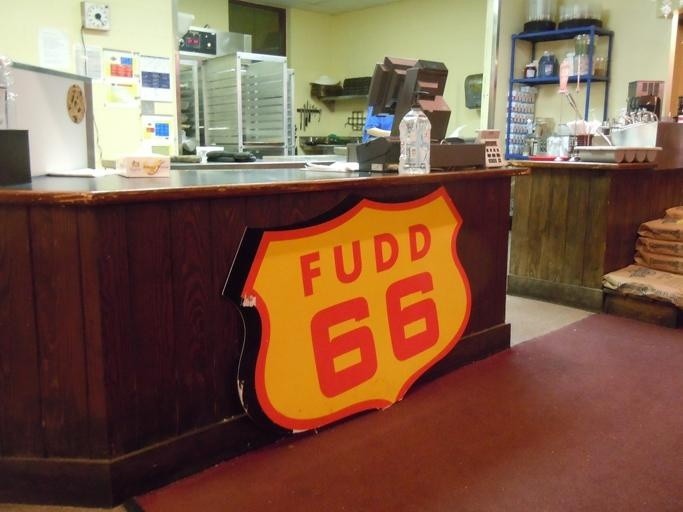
368, 56, 452, 144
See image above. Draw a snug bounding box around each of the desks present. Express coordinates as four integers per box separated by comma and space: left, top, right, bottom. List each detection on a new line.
506, 158, 681, 312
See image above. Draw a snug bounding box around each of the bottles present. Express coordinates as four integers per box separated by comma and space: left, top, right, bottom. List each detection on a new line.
399, 91, 432, 176
538, 51, 556, 77
564, 53, 578, 76
593, 56, 606, 77
523, 143, 530, 155
568, 136, 578, 157
573, 34, 599, 76
602, 121, 610, 135
524, 64, 536, 78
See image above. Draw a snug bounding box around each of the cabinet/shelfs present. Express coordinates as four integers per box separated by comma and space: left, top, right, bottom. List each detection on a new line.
505, 24, 614, 160
